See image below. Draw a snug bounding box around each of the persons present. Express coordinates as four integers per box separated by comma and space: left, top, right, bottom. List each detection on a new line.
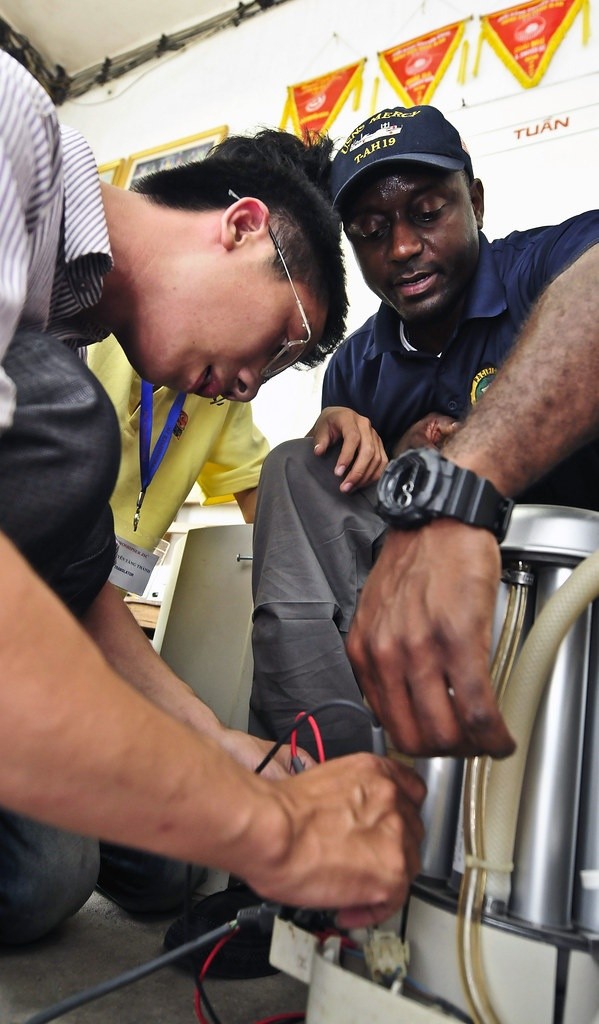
0, 48, 428, 930
1, 129, 386, 949
165, 108, 599, 981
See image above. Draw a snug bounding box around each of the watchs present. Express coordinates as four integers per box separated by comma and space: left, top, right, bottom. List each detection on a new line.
376, 447, 514, 544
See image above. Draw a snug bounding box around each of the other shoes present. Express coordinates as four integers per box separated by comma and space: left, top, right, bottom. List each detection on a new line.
164, 872, 334, 978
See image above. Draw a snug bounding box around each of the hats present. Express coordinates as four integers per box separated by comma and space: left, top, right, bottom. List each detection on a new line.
327, 105, 474, 213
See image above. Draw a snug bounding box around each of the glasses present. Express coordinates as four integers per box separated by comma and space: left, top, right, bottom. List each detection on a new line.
210, 189, 311, 406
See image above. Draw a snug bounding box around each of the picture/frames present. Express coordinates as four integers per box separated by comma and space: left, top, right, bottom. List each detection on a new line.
117, 125, 229, 192
98, 158, 124, 189
